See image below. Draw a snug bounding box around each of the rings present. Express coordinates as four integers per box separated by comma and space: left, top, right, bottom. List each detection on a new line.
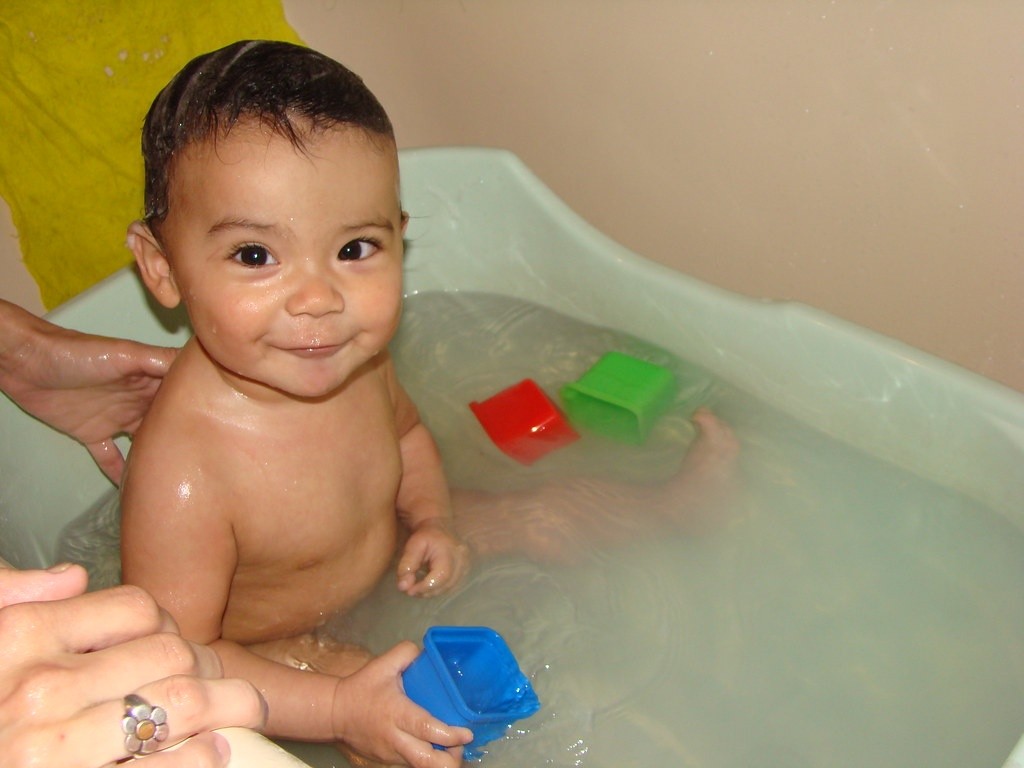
123, 694, 168, 759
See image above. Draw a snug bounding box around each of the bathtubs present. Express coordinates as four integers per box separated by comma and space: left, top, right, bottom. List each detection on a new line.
2, 145, 1024, 766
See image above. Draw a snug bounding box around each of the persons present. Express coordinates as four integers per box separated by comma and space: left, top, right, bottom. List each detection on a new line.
0, 301, 268, 768
117, 40, 472, 768
